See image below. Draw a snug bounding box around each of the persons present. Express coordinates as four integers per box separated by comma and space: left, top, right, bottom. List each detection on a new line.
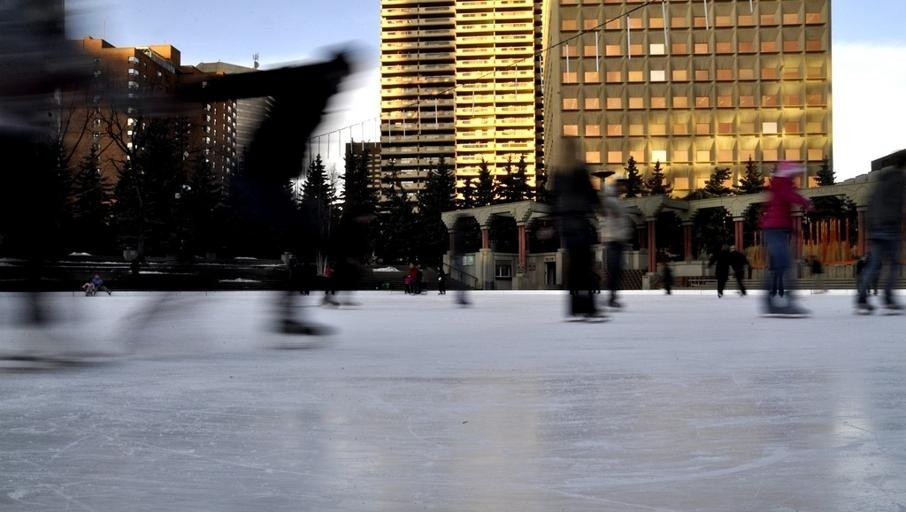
0, 0, 207, 336
90, 272, 104, 296
403, 262, 447, 297
322, 261, 337, 294
706, 163, 906, 325
545, 132, 626, 325
151, 31, 358, 341
82, 279, 95, 296
662, 265, 674, 295
